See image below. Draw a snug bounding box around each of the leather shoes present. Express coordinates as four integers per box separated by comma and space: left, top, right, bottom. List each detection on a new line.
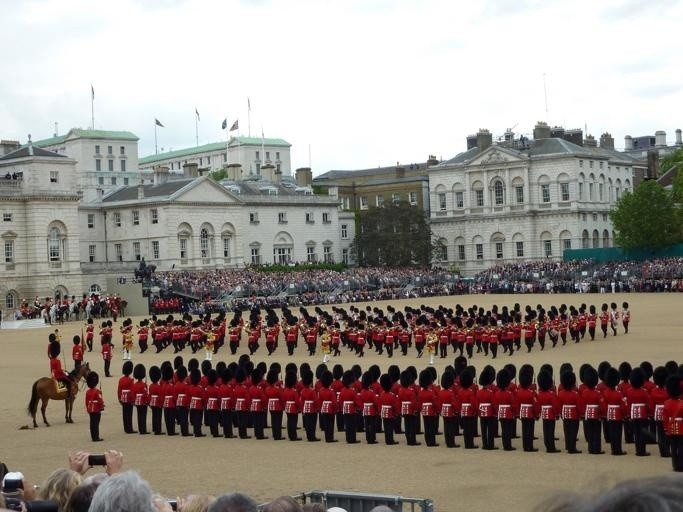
124, 431, 206, 437
213, 433, 338, 443
176, 423, 301, 429
338, 427, 520, 451
524, 437, 671, 457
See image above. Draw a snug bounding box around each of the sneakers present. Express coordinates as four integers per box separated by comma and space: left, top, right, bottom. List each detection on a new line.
106, 374, 112, 377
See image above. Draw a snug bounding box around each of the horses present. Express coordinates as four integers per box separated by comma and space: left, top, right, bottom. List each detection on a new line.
16, 300, 129, 325
135, 265, 156, 283
27, 361, 91, 428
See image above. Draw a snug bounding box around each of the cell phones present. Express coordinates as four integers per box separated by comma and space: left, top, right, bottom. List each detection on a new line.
88, 455, 106, 465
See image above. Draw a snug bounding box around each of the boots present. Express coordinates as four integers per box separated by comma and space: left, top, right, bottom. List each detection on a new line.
123, 350, 131, 360
205, 352, 213, 361
91, 431, 103, 441
429, 353, 434, 364
323, 354, 330, 362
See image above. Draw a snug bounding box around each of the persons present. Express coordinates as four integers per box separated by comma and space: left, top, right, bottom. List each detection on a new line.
72, 335, 83, 368
1, 449, 682, 511
86, 318, 94, 352
98, 320, 114, 377
118, 356, 682, 472
20, 293, 121, 324
119, 318, 135, 359
133, 257, 682, 364
85, 371, 105, 442
47, 334, 73, 399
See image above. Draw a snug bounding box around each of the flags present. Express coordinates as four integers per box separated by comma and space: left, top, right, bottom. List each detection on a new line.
156, 120, 164, 127
222, 120, 226, 129
229, 120, 238, 131
196, 109, 201, 121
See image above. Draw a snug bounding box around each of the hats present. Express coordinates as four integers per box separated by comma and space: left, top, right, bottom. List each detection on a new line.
73, 335, 80, 345
152, 312, 225, 328
87, 318, 93, 324
49, 334, 56, 343
149, 366, 161, 383
102, 320, 112, 327
286, 362, 682, 389
666, 375, 681, 400
140, 319, 149, 326
134, 364, 145, 379
122, 361, 133, 374
87, 372, 98, 388
161, 356, 281, 385
231, 303, 628, 329
123, 318, 131, 327
50, 341, 60, 359
